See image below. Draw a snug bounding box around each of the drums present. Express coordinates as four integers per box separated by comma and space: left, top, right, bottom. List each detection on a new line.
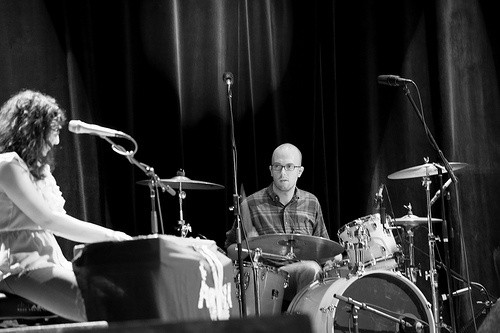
229, 258, 291, 319
336, 212, 403, 268
286, 269, 437, 333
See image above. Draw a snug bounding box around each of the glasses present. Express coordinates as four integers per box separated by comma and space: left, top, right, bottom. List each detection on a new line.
269, 163, 303, 172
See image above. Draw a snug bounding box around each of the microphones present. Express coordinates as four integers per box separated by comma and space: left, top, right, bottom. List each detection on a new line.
394, 226, 419, 244
378, 74, 413, 87
223, 72, 234, 85
372, 184, 385, 214
68, 120, 130, 140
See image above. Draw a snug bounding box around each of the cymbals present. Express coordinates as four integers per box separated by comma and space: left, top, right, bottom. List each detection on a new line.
387, 160, 470, 180
135, 176, 225, 191
241, 233, 346, 261
389, 215, 443, 228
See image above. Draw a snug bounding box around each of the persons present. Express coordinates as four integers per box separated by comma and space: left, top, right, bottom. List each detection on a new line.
0, 90, 133, 321
240, 143, 342, 292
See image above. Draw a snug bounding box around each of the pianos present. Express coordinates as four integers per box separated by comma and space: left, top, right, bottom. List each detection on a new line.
71, 234, 233, 321
